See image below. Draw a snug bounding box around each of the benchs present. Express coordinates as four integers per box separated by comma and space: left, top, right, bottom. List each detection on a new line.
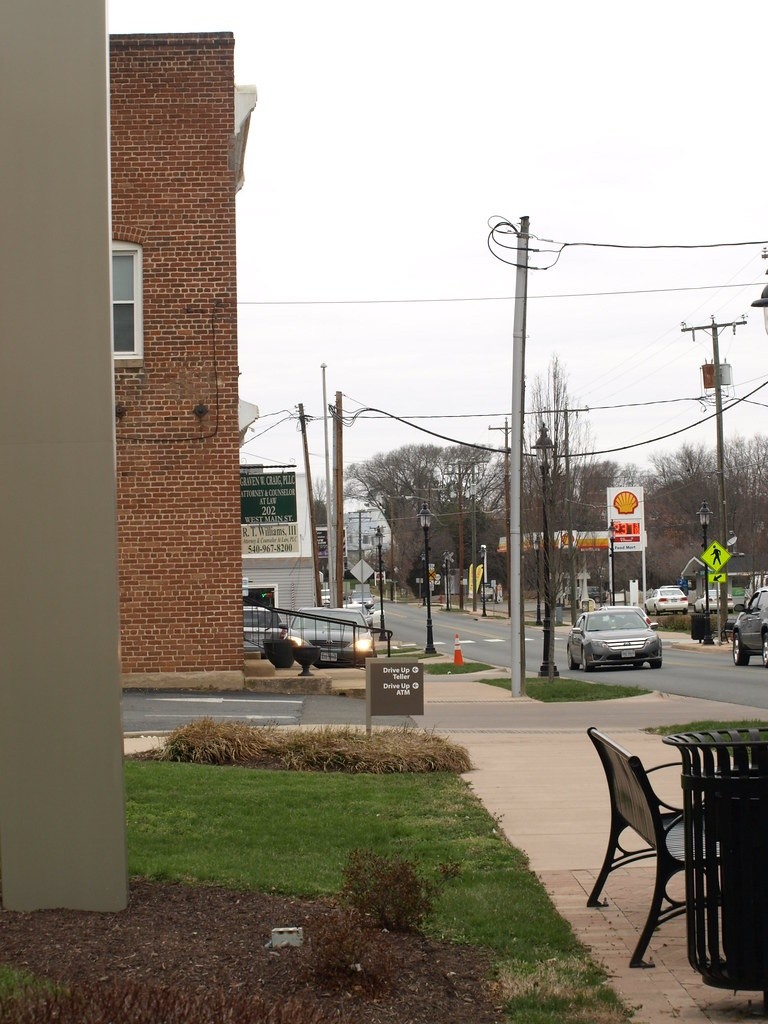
587, 728, 727, 969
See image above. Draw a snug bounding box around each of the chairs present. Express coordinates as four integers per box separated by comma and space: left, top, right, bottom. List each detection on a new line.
720, 618, 737, 644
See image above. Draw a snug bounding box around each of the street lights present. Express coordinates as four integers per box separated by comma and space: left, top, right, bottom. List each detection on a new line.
372, 526, 388, 642
478, 547, 487, 615
416, 502, 437, 653
607, 519, 615, 607
530, 421, 560, 677
419, 549, 427, 605
696, 499, 714, 644
443, 550, 451, 611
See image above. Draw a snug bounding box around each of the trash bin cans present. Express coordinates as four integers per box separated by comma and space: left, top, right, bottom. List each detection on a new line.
690, 614, 705, 640
661, 727, 768, 990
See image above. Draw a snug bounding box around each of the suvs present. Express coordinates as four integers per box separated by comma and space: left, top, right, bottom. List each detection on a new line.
564, 586, 608, 603
730, 586, 767, 667
480, 584, 494, 601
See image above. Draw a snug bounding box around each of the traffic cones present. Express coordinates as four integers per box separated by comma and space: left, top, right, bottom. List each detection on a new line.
453, 634, 464, 666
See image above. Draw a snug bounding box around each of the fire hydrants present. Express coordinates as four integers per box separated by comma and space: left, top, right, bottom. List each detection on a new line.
439, 595, 442, 603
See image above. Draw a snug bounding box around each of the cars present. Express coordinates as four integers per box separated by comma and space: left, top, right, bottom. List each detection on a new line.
283, 606, 376, 668
645, 585, 688, 616
694, 590, 733, 615
244, 606, 288, 658
567, 606, 663, 672
343, 591, 375, 628
